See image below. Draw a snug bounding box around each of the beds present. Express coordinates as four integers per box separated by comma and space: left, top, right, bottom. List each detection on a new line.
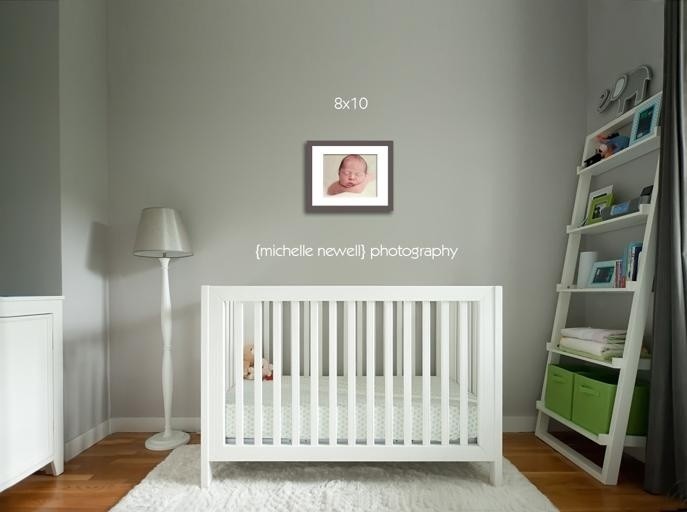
199, 286, 504, 491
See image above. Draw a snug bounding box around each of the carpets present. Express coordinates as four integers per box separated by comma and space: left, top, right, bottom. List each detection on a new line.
109, 443, 559, 512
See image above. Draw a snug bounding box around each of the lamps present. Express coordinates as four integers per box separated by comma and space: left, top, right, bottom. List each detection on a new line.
132, 206, 194, 451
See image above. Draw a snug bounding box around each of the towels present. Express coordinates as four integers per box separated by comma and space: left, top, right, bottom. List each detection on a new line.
559, 337, 624, 356
560, 326, 627, 343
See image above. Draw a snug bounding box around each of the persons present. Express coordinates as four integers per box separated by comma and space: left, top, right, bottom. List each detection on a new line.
323, 154, 375, 194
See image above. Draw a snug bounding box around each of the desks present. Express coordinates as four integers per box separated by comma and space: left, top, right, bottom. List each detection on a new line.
0, 294, 66, 493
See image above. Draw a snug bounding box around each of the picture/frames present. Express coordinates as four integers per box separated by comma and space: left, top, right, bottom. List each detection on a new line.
585, 194, 614, 224
307, 140, 393, 213
586, 184, 614, 219
628, 92, 663, 146
586, 260, 616, 287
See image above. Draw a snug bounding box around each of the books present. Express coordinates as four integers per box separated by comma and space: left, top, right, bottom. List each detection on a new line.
611, 239, 641, 288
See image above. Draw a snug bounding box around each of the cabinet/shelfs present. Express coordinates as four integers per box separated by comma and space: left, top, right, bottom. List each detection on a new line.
534, 90, 665, 484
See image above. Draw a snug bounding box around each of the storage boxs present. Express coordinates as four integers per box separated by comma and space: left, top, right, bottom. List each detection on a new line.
545, 362, 590, 421
572, 364, 649, 436
